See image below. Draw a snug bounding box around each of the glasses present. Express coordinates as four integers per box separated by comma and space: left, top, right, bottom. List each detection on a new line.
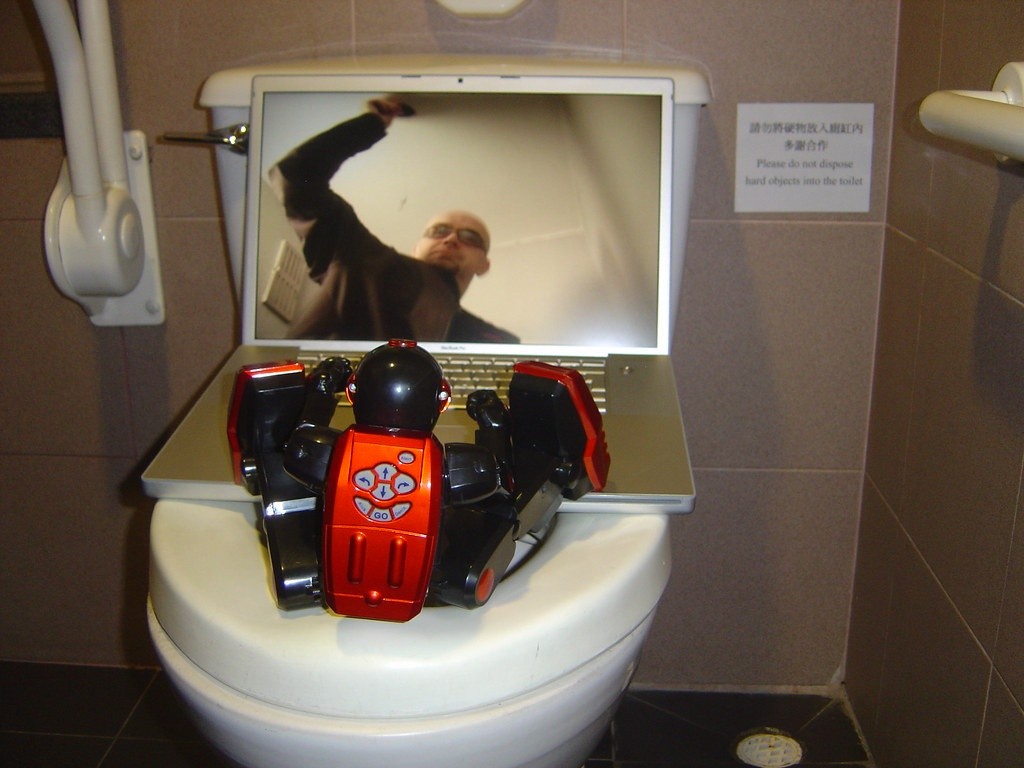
425, 224, 487, 255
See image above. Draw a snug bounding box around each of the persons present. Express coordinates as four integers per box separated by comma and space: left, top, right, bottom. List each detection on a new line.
228, 345, 608, 624
264, 101, 518, 344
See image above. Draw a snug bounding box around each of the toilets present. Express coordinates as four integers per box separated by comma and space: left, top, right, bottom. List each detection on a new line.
139, 48, 715, 768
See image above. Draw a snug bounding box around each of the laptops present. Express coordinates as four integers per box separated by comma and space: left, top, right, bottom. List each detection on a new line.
143, 76, 698, 515
